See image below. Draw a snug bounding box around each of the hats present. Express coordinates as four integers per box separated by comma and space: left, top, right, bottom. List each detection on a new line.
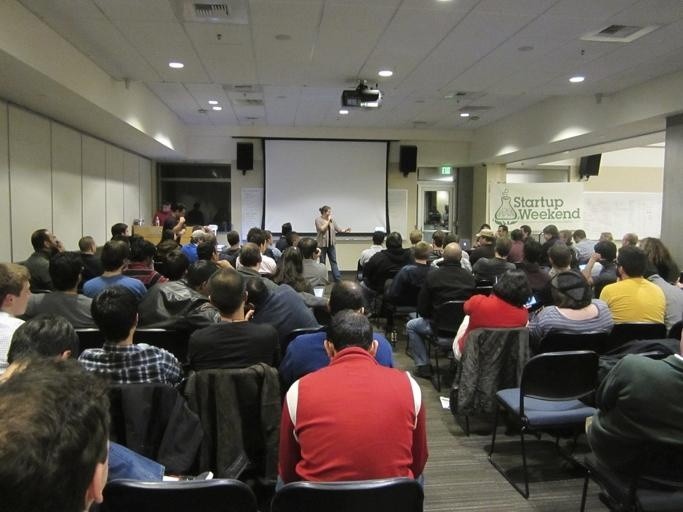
475, 229, 494, 238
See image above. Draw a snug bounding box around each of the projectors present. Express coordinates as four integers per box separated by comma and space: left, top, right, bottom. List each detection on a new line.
342, 90, 383, 108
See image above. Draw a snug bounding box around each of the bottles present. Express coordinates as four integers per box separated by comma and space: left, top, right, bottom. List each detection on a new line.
139, 215, 144, 227
390, 329, 398, 353
154, 216, 160, 227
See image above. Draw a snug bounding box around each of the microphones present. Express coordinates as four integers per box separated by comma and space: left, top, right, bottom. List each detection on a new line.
327, 214, 332, 223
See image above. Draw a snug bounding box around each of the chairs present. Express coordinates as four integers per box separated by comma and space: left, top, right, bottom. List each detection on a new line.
356, 251, 682, 511
0, 324, 426, 511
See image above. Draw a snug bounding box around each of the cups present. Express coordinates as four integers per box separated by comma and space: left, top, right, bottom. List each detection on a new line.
313, 286, 323, 297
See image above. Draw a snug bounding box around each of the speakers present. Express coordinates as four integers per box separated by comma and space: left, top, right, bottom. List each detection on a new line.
400, 146, 417, 172
579, 153, 602, 176
237, 143, 253, 170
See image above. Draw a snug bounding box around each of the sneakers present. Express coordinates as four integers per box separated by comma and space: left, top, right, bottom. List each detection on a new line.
413, 365, 434, 376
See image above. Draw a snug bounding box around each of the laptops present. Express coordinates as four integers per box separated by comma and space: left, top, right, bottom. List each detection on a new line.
461, 239, 477, 251
